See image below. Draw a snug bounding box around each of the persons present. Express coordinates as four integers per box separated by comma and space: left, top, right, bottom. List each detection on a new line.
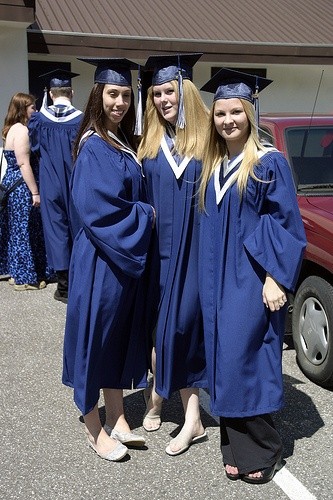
0, 92, 47, 291
136, 54, 211, 456
197, 66, 307, 485
26, 68, 86, 303
63, 56, 156, 461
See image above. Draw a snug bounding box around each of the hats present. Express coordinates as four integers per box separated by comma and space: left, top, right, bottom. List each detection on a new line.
38, 69, 79, 112
76, 56, 149, 136
201, 64, 273, 139
140, 51, 202, 129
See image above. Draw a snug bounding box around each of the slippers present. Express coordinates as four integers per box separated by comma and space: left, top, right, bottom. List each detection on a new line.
142, 388, 163, 432
165, 423, 206, 455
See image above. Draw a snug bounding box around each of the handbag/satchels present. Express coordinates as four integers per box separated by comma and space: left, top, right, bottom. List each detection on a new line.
0, 185, 7, 212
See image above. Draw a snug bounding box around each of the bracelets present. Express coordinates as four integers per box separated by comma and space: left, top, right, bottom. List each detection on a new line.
32, 194, 39, 195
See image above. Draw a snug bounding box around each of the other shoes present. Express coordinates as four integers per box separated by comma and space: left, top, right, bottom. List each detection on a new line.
54, 289, 70, 303
15, 279, 46, 290
87, 426, 128, 461
102, 423, 146, 447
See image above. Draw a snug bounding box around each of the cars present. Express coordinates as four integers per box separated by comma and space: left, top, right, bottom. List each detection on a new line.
258, 112, 333, 389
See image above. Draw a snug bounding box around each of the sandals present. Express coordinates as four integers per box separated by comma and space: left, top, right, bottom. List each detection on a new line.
242, 455, 283, 483
224, 463, 242, 480
